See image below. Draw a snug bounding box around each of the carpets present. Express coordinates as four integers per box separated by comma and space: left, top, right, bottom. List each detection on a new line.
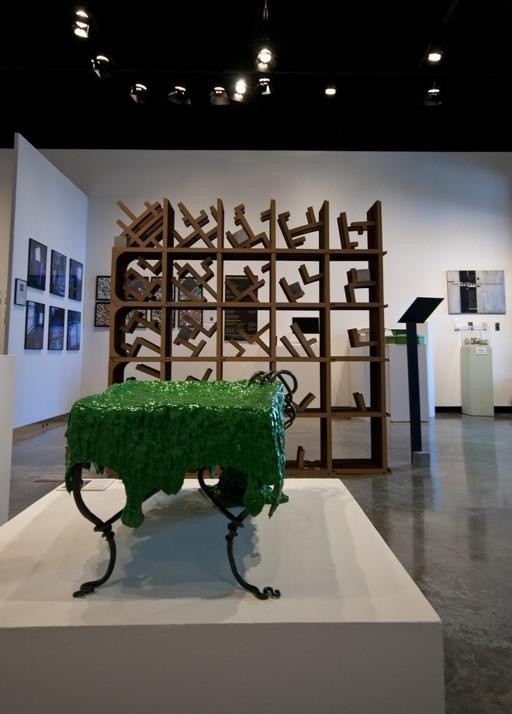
1, 479, 444, 628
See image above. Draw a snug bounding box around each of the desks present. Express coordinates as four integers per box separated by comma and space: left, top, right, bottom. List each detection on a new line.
69, 378, 288, 600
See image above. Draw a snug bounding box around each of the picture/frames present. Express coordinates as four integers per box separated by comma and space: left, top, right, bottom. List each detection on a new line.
96, 277, 204, 327
15, 238, 83, 350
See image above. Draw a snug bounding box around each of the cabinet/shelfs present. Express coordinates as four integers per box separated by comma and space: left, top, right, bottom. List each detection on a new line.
108, 200, 391, 474
